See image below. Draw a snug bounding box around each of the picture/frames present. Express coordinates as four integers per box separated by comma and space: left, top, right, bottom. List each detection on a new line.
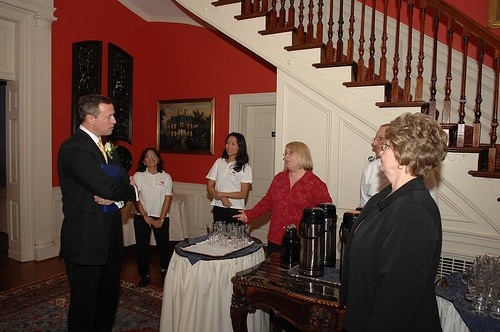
157, 98, 215, 155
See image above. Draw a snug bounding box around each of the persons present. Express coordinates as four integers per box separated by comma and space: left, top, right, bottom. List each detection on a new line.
206, 132, 253, 226
233, 142, 332, 256
131, 148, 174, 287
359, 123, 391, 207
56, 95, 139, 332
336, 111, 447, 332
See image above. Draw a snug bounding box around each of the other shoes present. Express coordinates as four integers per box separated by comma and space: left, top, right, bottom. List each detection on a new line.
161, 270, 167, 279
138, 276, 150, 287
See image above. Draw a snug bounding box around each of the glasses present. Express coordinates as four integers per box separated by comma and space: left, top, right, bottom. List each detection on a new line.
283, 152, 292, 156
381, 144, 394, 150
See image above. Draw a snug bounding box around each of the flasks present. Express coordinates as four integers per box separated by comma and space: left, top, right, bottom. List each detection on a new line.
299, 207, 325, 277
339, 207, 363, 280
316, 203, 338, 267
280, 228, 301, 270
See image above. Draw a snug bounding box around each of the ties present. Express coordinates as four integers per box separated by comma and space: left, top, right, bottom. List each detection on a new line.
97, 139, 108, 164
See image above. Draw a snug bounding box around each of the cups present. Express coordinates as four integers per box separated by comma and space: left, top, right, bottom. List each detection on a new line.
465, 252, 500, 319
206, 221, 251, 249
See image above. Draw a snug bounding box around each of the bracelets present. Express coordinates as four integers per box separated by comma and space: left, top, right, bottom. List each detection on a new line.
161, 220, 164, 222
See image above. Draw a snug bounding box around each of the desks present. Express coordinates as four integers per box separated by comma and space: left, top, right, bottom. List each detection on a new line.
434, 272, 500, 332
123, 193, 190, 246
159, 236, 271, 332
230, 253, 344, 332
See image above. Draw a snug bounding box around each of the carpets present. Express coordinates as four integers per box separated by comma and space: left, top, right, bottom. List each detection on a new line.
0, 271, 164, 332
